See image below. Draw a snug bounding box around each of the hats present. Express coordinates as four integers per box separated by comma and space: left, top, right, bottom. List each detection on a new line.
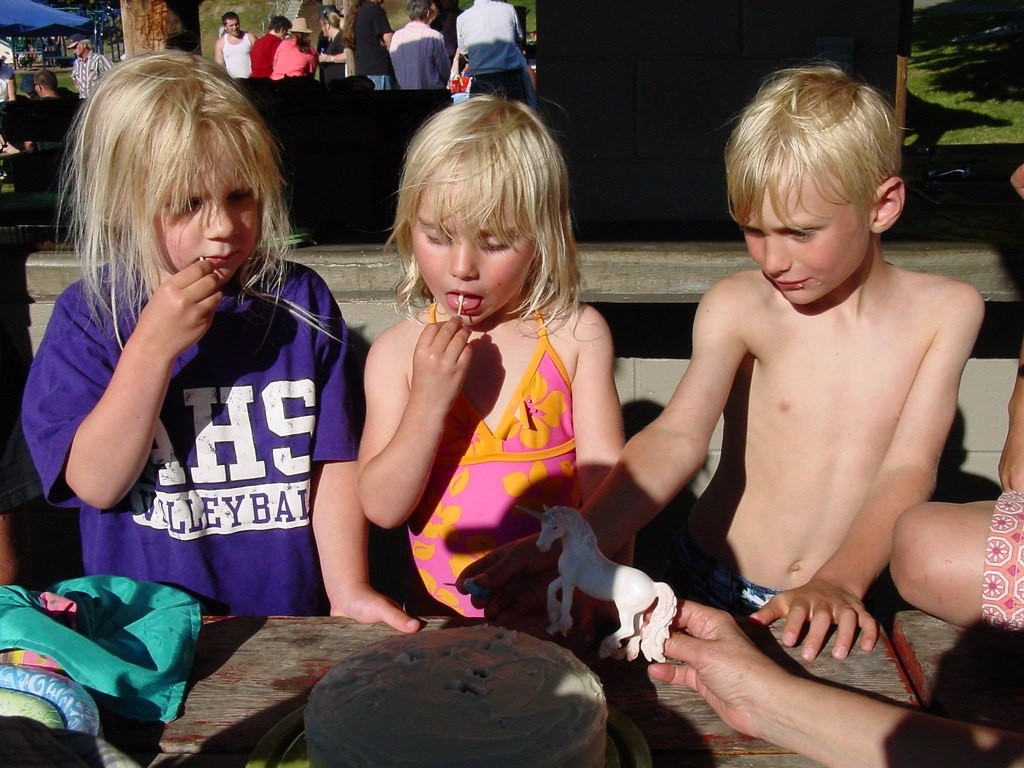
66, 34, 89, 50
289, 17, 313, 33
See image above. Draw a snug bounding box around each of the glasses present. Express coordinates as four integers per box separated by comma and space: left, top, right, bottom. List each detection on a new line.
32, 81, 41, 88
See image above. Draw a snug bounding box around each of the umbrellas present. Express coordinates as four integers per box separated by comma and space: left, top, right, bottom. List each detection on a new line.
0, 0, 94, 69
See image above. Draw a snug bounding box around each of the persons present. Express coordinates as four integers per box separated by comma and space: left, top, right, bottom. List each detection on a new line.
215, 11, 256, 79
21, 51, 420, 634
649, 600, 1024, 768
1, 229, 35, 584
271, 18, 318, 80
389, 1, 451, 89
342, 0, 395, 89
249, 16, 293, 77
357, 93, 635, 644
456, 1, 536, 108
0, 37, 116, 183
889, 165, 1024, 633
316, 5, 346, 81
455, 59, 985, 663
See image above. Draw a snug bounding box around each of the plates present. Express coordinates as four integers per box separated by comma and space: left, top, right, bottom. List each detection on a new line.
247, 702, 652, 768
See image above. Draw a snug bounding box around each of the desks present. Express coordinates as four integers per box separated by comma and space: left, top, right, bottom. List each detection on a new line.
104, 610, 1024, 768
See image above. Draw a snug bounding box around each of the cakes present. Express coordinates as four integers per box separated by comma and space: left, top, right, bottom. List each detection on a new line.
304, 624, 610, 768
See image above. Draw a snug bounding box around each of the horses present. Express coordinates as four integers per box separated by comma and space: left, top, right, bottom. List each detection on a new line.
516, 501, 678, 664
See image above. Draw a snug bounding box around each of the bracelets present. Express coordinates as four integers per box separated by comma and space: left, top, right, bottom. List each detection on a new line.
332, 56, 333, 62
